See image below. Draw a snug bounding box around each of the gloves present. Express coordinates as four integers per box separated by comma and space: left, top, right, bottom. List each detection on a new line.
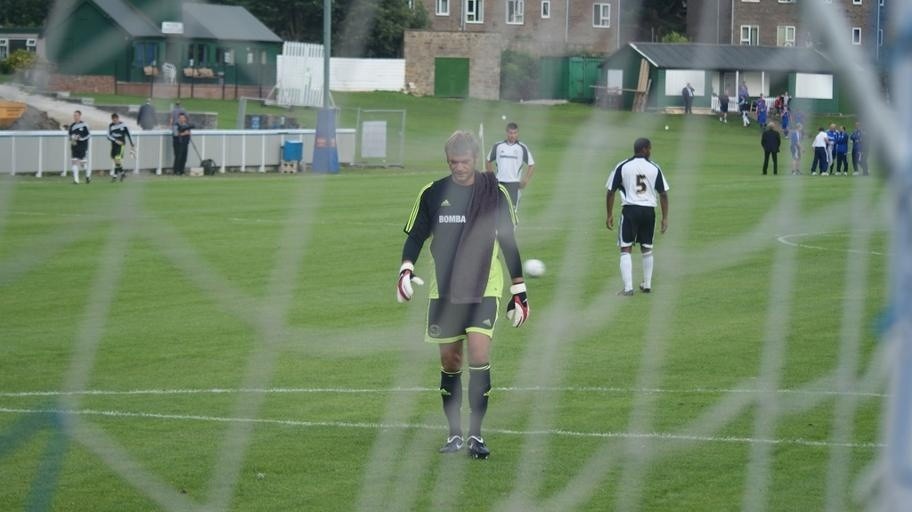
396, 263, 425, 303
506, 281, 530, 329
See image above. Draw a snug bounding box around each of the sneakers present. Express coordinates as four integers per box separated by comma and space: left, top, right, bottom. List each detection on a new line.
439, 436, 465, 453
639, 283, 652, 293
812, 171, 860, 176
617, 289, 634, 297
467, 435, 490, 460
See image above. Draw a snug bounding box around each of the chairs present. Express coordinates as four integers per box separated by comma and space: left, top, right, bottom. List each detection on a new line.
144, 64, 219, 84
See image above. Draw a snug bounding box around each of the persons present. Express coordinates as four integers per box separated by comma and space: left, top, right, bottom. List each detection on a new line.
109, 113, 134, 183
715, 81, 888, 177
66, 109, 92, 183
171, 100, 191, 128
604, 136, 670, 296
138, 97, 160, 131
682, 83, 695, 116
173, 112, 192, 175
483, 120, 536, 241
395, 129, 530, 460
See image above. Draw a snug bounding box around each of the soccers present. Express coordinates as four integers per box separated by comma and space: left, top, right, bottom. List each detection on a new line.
501, 115, 506, 120
664, 125, 668, 131
524, 259, 545, 277
